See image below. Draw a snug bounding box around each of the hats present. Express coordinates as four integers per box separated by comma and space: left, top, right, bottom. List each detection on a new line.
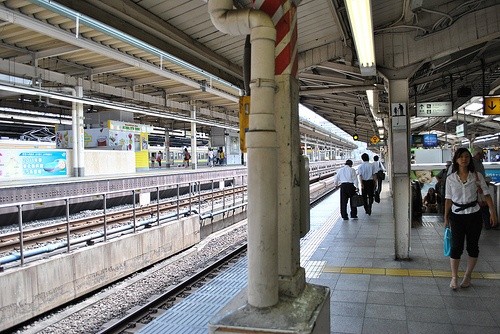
445, 161, 451, 166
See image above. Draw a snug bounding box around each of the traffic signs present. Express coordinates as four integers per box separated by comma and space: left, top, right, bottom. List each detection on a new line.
417, 102, 453, 117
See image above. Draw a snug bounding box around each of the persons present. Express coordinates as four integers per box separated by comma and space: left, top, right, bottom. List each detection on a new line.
156, 151, 163, 168
358, 153, 377, 216
469, 145, 499, 230
413, 170, 439, 199
182, 147, 190, 167
424, 187, 438, 213
444, 148, 498, 289
206, 146, 225, 167
150, 152, 156, 168
372, 154, 386, 204
334, 160, 359, 221
435, 160, 453, 215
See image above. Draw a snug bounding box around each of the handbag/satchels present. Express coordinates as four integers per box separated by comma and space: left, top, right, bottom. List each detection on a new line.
377, 170, 385, 180
443, 225, 451, 257
475, 171, 487, 206
352, 192, 364, 207
375, 191, 380, 203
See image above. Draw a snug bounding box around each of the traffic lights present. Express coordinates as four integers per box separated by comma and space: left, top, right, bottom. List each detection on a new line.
353, 135, 358, 140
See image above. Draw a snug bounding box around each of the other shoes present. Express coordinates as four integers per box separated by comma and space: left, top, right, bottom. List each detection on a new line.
460, 282, 472, 288
344, 217, 348, 220
450, 280, 457, 289
366, 205, 371, 216
351, 215, 358, 218
